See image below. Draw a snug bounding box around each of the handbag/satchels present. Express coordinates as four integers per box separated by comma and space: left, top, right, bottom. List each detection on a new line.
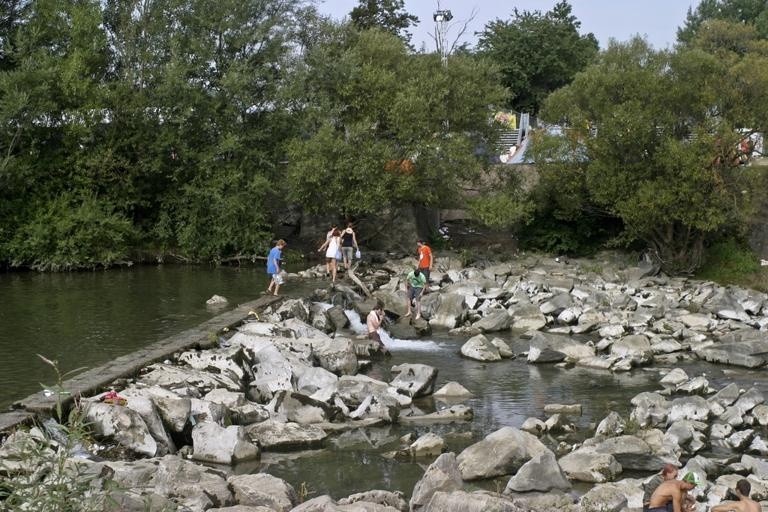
272, 273, 284, 285
382, 318, 390, 331
355, 251, 361, 258
336, 249, 342, 261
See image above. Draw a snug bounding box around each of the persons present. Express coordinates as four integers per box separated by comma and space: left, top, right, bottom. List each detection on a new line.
265, 239, 287, 297
648, 471, 697, 511
340, 221, 359, 270
323, 222, 343, 273
438, 224, 453, 242
367, 305, 391, 355
711, 478, 761, 512
415, 239, 433, 291
642, 463, 678, 511
404, 268, 427, 320
317, 229, 345, 283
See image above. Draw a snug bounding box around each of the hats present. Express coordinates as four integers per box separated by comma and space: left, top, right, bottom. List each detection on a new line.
683, 471, 702, 485
